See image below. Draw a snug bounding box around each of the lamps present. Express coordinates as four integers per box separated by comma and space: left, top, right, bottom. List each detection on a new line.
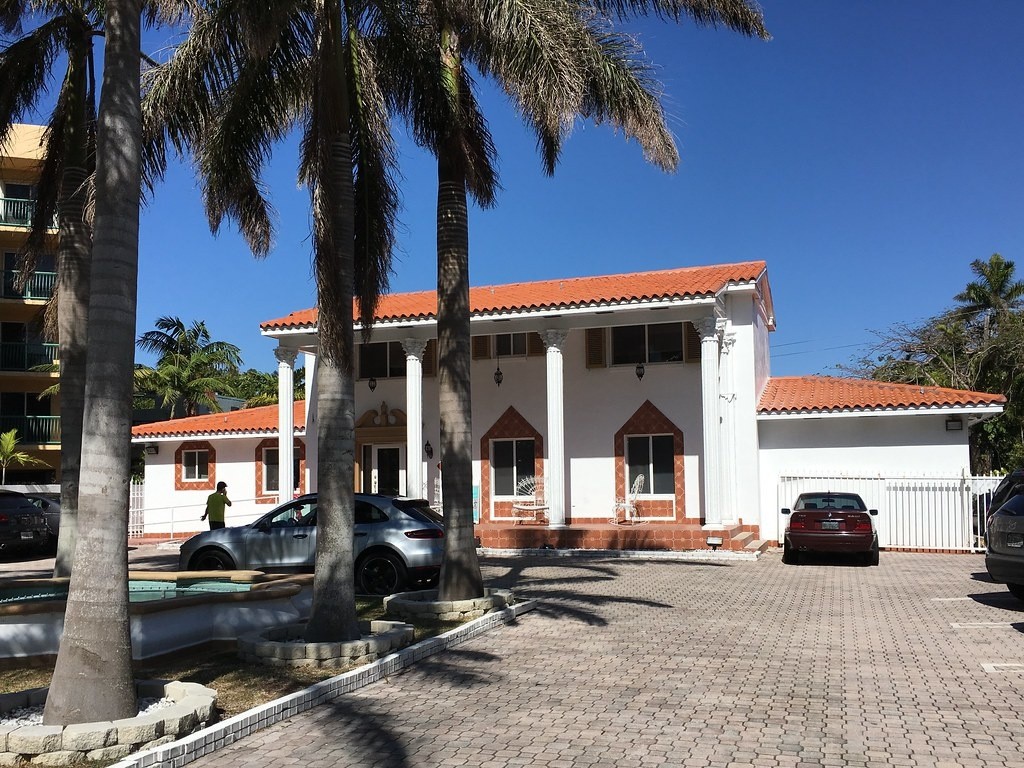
424, 440, 434, 459
144, 443, 159, 457
494, 334, 503, 387
946, 415, 962, 431
635, 324, 645, 382
706, 536, 723, 552
367, 343, 379, 393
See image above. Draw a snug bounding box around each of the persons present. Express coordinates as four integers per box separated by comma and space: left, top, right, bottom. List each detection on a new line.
200, 482, 232, 530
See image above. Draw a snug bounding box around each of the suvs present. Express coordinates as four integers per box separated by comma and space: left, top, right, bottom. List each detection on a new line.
177, 492, 446, 594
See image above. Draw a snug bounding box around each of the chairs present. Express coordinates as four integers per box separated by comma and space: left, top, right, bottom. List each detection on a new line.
606, 474, 649, 528
510, 474, 550, 526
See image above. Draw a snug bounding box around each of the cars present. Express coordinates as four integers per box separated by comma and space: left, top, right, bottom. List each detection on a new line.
985, 493, 1024, 594
780, 491, 892, 563
0, 489, 48, 558
27, 492, 61, 539
